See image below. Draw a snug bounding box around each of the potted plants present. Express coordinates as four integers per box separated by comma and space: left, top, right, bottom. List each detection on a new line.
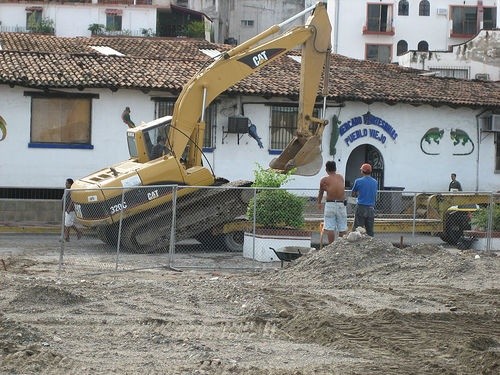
243, 161, 313, 264
463, 202, 500, 251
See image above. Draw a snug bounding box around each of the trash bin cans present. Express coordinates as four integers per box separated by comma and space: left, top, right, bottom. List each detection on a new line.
383, 187, 405, 214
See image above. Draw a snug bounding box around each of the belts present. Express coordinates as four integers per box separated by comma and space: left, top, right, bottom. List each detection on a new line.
327, 200, 344, 202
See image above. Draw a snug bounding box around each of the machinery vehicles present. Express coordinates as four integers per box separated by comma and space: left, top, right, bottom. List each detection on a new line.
68, 1, 333, 255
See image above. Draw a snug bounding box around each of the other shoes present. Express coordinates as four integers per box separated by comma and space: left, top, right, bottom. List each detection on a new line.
64, 237, 69, 241
77, 231, 82, 239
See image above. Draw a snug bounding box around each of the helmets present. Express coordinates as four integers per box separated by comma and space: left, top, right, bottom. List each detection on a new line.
360, 164, 372, 173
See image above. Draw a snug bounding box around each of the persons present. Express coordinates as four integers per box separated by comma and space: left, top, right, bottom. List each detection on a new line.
59, 178, 82, 242
448, 173, 462, 192
317, 161, 348, 244
351, 164, 378, 237
